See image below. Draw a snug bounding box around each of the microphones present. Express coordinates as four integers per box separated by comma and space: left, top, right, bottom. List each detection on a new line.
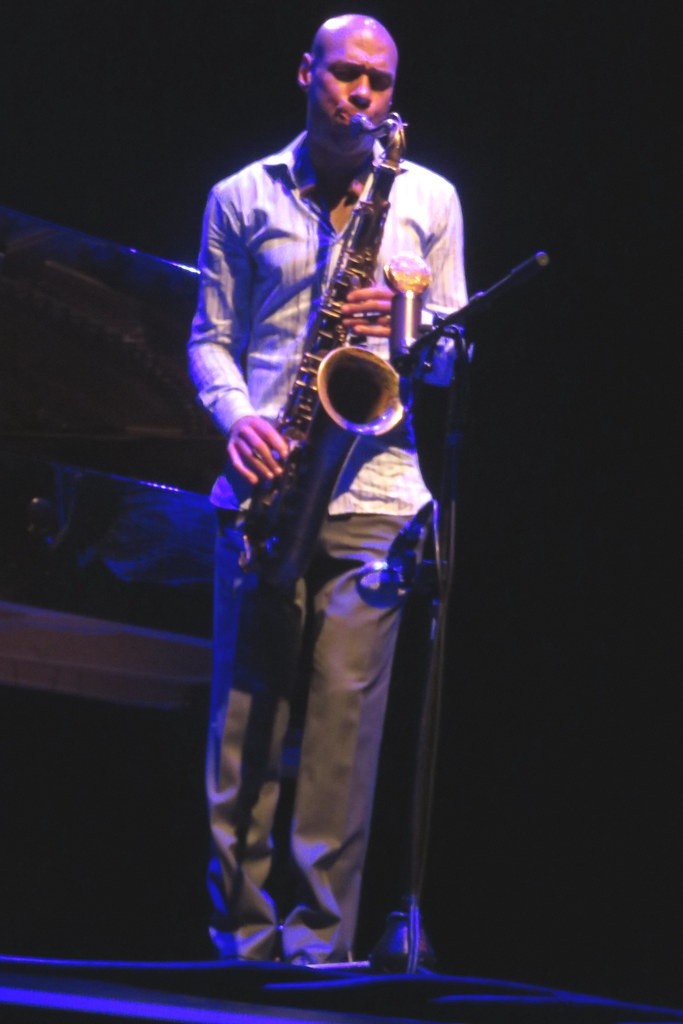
382, 249, 431, 368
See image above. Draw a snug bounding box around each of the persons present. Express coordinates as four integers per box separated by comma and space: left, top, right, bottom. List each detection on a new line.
178, 9, 472, 972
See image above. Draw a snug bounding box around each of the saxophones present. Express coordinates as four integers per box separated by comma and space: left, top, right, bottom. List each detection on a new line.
239, 108, 417, 589
